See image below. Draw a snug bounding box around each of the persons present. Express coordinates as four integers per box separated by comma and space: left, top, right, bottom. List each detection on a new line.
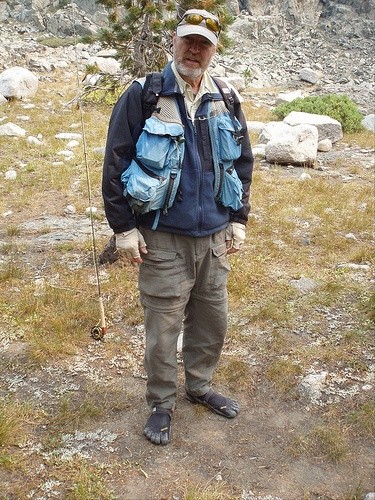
102, 8, 254, 445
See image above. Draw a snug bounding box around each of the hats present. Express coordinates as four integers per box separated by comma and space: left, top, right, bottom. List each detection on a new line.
177, 9, 221, 45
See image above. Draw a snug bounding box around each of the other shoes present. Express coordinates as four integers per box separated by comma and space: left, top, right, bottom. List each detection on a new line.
143, 407, 172, 444
187, 388, 239, 418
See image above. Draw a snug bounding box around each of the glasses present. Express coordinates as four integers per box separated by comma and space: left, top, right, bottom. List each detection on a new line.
178, 13, 221, 37
